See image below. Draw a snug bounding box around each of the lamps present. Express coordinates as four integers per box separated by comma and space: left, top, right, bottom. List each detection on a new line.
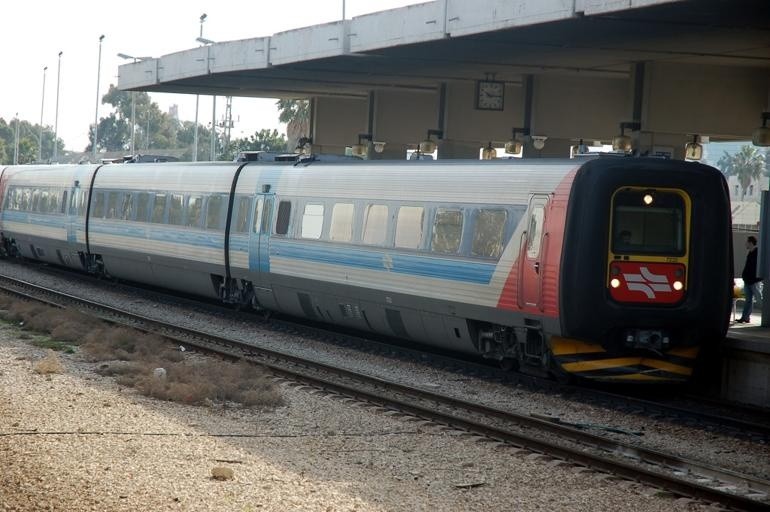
505, 127, 530, 154
531, 135, 547, 149
572, 139, 589, 156
294, 138, 312, 153
686, 135, 703, 160
752, 112, 770, 147
352, 134, 372, 157
612, 122, 641, 152
373, 142, 385, 152
420, 129, 443, 153
483, 142, 496, 159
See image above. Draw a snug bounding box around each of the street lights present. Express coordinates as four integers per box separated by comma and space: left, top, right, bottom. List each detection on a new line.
93, 35, 106, 153
117, 53, 152, 155
54, 52, 64, 160
38, 67, 48, 160
192, 13, 207, 161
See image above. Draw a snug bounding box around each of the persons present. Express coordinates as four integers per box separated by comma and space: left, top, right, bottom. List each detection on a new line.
735, 234, 766, 323
613, 229, 645, 255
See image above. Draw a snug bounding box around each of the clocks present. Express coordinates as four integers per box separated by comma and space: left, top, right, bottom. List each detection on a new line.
473, 80, 505, 112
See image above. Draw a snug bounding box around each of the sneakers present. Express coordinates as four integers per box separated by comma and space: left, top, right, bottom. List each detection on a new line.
735, 318, 750, 323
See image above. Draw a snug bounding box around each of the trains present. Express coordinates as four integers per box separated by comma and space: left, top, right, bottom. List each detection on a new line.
0, 152, 734, 389
